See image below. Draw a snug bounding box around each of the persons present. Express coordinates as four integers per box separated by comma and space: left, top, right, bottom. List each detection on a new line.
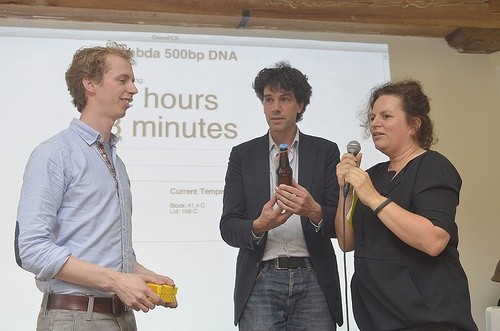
334, 80, 478, 331
219, 60, 343, 331
14, 40, 178, 331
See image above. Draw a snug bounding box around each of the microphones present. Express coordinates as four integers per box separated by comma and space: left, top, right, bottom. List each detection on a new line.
343, 141, 361, 197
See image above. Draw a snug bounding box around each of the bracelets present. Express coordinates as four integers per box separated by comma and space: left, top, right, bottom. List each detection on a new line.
374, 197, 393, 217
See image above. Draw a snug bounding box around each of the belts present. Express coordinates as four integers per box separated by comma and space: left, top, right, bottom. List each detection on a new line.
273, 257, 312, 270
40, 293, 130, 316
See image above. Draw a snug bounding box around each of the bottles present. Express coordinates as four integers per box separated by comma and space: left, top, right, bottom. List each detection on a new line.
275, 144, 293, 186
